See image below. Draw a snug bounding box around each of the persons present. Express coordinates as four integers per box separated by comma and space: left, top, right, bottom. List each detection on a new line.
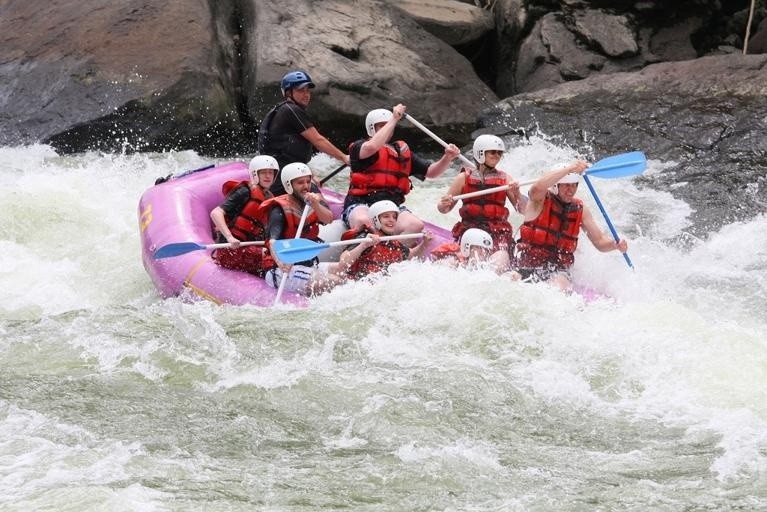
209, 154, 280, 279
256, 162, 333, 272
340, 200, 433, 282
342, 104, 461, 248
438, 135, 521, 262
518, 161, 627, 283
258, 71, 351, 196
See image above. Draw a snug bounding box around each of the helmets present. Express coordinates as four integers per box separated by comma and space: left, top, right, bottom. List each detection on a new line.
281, 71, 313, 99
249, 156, 281, 186
548, 163, 580, 197
368, 200, 401, 232
366, 109, 394, 139
281, 162, 313, 196
473, 135, 507, 166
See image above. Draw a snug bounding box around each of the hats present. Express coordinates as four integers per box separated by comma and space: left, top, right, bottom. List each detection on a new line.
293, 82, 317, 92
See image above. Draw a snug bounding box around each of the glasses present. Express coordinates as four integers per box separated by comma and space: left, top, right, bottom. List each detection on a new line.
487, 151, 503, 156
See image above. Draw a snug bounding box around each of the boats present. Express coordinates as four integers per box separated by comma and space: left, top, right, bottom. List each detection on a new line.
137, 160, 602, 306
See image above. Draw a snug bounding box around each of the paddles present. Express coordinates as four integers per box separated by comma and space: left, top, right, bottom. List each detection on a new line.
270, 232, 425, 265
152, 240, 265, 260
453, 150, 647, 200
